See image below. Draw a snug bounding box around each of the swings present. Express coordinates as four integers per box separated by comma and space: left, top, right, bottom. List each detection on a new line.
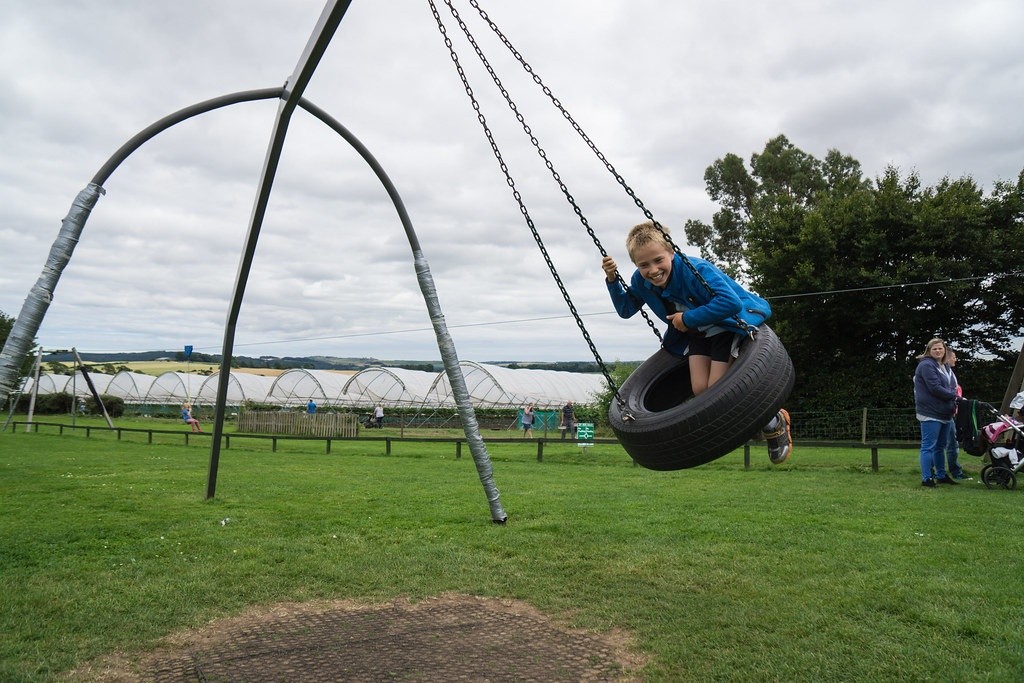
424, 0, 795, 470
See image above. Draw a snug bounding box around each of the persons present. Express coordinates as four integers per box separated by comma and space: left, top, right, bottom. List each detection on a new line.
522, 403, 534, 438
562, 400, 577, 438
77, 401, 87, 415
182, 403, 202, 432
601, 222, 792, 464
374, 403, 384, 429
307, 399, 316, 413
931, 348, 970, 479
914, 338, 960, 488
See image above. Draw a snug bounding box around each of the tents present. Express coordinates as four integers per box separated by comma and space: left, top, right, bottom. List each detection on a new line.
12, 361, 618, 425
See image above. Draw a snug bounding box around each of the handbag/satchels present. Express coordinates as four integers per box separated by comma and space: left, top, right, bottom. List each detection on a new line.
531, 416, 535, 424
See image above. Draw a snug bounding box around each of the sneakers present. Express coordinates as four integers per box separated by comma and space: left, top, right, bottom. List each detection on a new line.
763, 408, 792, 464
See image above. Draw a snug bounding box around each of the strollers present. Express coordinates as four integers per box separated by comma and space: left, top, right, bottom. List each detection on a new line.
363, 415, 376, 428
953, 399, 1024, 491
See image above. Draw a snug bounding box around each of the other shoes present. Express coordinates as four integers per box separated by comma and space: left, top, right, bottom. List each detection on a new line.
922, 477, 935, 488
937, 474, 958, 484
953, 472, 972, 480
